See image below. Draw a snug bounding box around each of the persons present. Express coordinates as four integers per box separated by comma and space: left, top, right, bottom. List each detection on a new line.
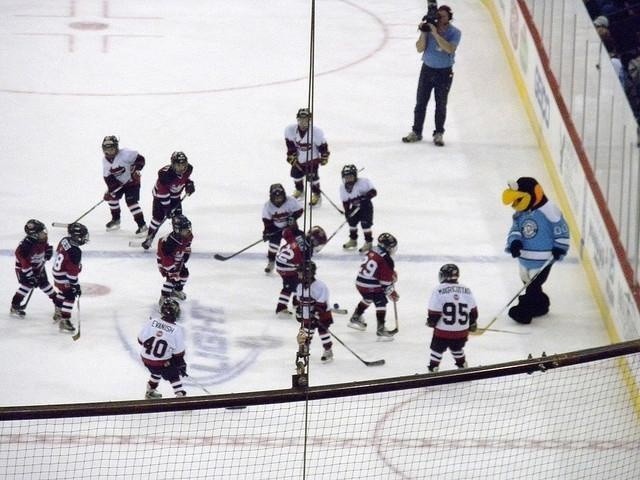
156, 213, 194, 314
422, 264, 479, 372
288, 262, 335, 366
345, 231, 401, 339
262, 183, 302, 277
100, 134, 149, 238
583, 0, 640, 124
54, 222, 90, 335
284, 108, 331, 209
402, 4, 462, 148
273, 223, 328, 318
9, 218, 62, 323
141, 151, 195, 252
339, 163, 377, 254
135, 298, 190, 399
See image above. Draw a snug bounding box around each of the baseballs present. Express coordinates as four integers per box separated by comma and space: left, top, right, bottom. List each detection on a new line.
333, 302, 338, 309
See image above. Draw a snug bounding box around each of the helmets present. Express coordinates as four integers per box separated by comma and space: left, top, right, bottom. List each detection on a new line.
101, 134, 119, 149
159, 298, 182, 319
295, 260, 318, 275
341, 163, 358, 177
441, 263, 460, 282
67, 222, 89, 237
170, 151, 188, 164
172, 214, 192, 229
23, 218, 46, 236
269, 183, 285, 194
378, 232, 398, 248
308, 224, 328, 245
296, 107, 312, 119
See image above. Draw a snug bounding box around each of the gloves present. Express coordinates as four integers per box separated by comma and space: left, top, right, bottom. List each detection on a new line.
27, 274, 40, 286
387, 287, 401, 303
44, 245, 53, 260
185, 182, 196, 196
310, 312, 321, 325
286, 152, 299, 166
262, 229, 274, 242
320, 152, 331, 165
181, 252, 190, 263
71, 285, 82, 298
130, 170, 141, 185
286, 216, 296, 227
104, 192, 118, 201
164, 205, 178, 219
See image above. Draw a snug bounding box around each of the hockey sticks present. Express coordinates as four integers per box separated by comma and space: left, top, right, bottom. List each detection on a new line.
52, 186, 124, 229
129, 193, 186, 248
383, 284, 399, 337
214, 226, 290, 261
73, 295, 81, 341
311, 206, 360, 253
15, 259, 46, 310
327, 328, 385, 366
294, 161, 347, 215
185, 375, 248, 410
467, 254, 554, 335
330, 309, 348, 314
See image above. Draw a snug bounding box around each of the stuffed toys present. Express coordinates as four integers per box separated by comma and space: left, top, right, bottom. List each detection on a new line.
501, 176, 571, 325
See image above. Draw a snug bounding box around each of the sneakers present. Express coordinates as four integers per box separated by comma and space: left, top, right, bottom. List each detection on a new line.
10, 306, 26, 316
320, 351, 334, 362
264, 262, 276, 273
402, 129, 423, 144
349, 316, 368, 328
135, 224, 156, 250
275, 308, 293, 315
158, 288, 188, 304
432, 132, 446, 147
145, 390, 163, 400
377, 328, 394, 337
290, 189, 322, 206
342, 239, 373, 252
105, 219, 121, 228
53, 311, 76, 331
